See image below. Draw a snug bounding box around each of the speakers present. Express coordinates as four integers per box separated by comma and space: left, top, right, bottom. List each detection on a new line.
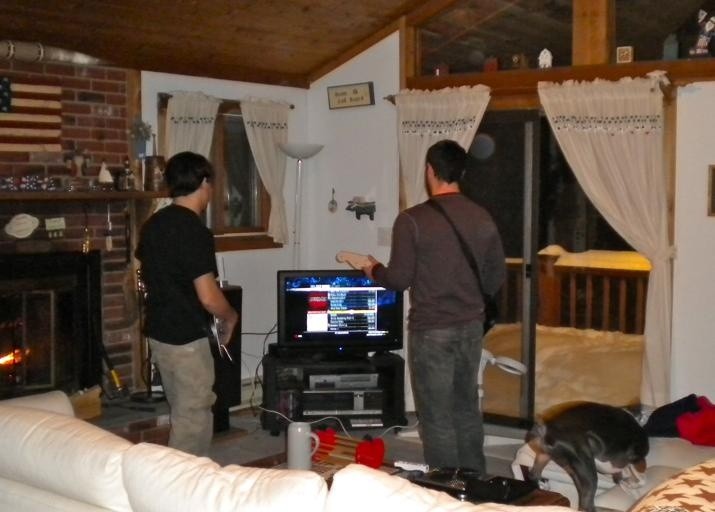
216, 287, 241, 406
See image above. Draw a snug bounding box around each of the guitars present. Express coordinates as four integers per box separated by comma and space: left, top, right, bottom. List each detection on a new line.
336, 250, 500, 336
208, 314, 222, 359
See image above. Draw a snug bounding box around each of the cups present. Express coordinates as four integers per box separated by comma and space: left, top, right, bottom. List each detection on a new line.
287, 422, 320, 471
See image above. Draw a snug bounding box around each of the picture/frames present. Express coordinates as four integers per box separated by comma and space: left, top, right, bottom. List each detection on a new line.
327, 82, 375, 110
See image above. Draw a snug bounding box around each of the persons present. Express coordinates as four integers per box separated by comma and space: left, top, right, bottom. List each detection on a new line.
361, 139, 508, 473
133, 148, 238, 455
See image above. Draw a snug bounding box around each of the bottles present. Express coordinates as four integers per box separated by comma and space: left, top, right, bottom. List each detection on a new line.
118, 157, 135, 192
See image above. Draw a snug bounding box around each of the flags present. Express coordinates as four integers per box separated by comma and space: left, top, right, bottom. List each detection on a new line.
0, 75, 63, 153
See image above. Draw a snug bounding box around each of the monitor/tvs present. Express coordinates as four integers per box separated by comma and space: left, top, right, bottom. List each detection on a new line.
278, 269, 402, 362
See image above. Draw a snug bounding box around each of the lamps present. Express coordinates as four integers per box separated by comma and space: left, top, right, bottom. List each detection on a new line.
279, 142, 325, 270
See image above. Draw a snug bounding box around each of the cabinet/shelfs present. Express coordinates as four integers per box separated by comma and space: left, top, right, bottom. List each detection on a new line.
262, 352, 409, 436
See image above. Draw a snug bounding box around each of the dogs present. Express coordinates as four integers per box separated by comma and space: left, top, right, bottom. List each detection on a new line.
524, 399, 650, 512
